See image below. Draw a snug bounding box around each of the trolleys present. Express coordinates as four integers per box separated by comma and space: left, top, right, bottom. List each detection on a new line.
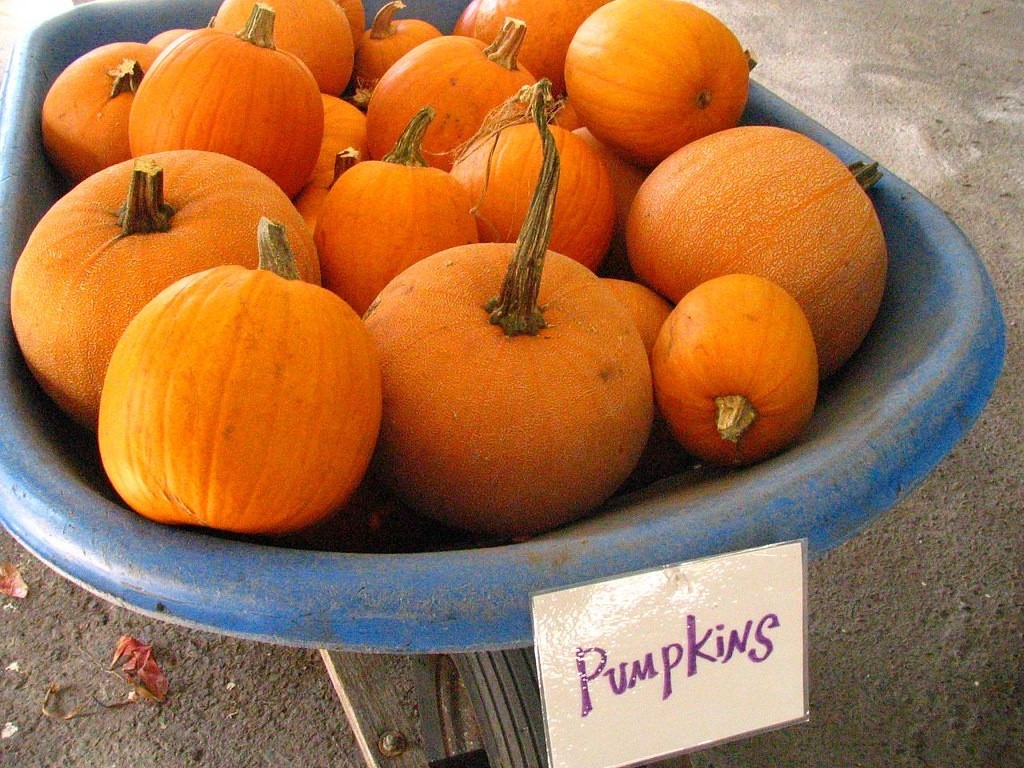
0, 0, 1006, 768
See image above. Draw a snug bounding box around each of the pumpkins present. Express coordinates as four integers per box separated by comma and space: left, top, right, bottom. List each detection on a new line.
11, 0, 890, 535
